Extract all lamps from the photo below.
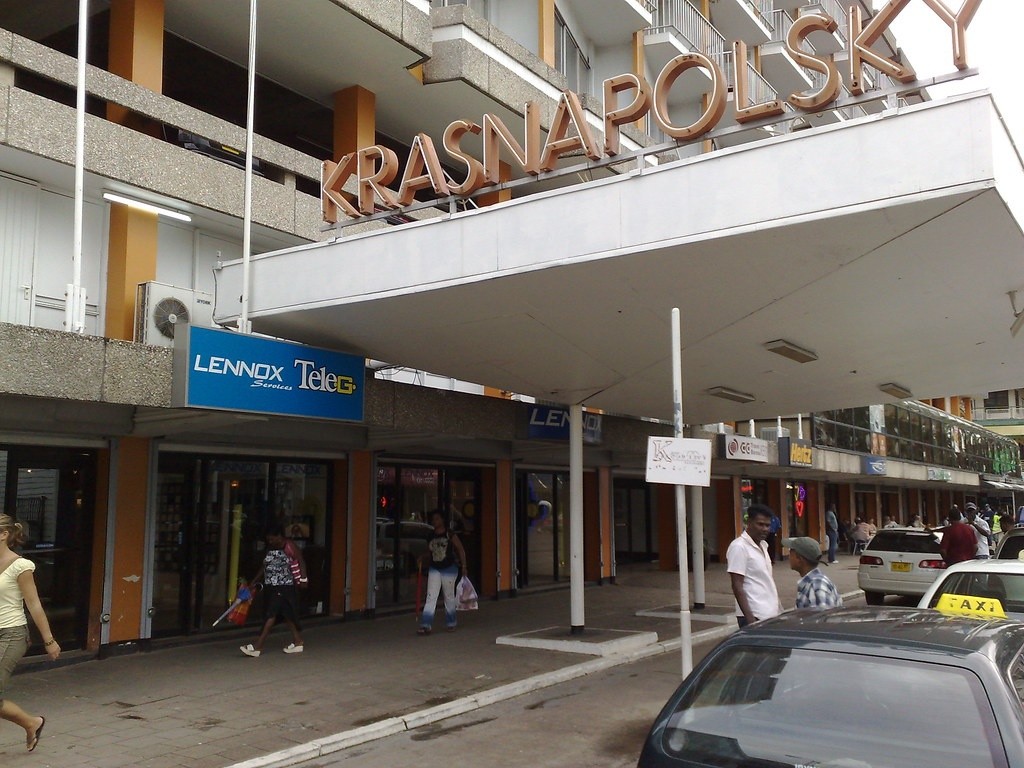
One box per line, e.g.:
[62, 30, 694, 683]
[707, 387, 756, 403]
[879, 383, 913, 399]
[764, 341, 817, 363]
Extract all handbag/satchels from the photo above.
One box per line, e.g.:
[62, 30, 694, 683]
[455, 577, 478, 610]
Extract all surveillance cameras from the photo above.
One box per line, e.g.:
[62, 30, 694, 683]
[1011, 308, 1024, 337]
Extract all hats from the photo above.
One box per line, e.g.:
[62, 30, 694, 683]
[964, 502, 976, 511]
[781, 537, 822, 563]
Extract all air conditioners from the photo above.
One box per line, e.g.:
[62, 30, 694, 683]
[760, 427, 791, 441]
[132, 280, 213, 349]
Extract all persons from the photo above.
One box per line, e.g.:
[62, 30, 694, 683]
[979, 502, 1004, 548]
[248, 526, 309, 659]
[727, 503, 784, 629]
[959, 501, 991, 561]
[937, 507, 979, 567]
[781, 537, 844, 610]
[824, 501, 839, 564]
[415, 510, 468, 637]
[912, 514, 929, 528]
[0, 510, 62, 754]
[883, 516, 900, 528]
[848, 515, 876, 554]
[998, 514, 1015, 534]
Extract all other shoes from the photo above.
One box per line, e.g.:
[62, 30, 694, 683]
[831, 561, 839, 564]
[416, 627, 431, 635]
[447, 626, 455, 633]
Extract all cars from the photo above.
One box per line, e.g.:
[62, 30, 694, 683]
[914, 523, 1024, 625]
[857, 525, 943, 606]
[637, 592, 1024, 768]
[374, 515, 434, 578]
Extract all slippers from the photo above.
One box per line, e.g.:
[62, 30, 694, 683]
[283, 643, 303, 653]
[27, 716, 44, 752]
[240, 644, 260, 657]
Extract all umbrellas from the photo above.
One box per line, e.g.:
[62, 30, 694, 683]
[212, 582, 264, 627]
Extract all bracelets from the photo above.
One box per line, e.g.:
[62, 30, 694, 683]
[299, 576, 307, 582]
[462, 564, 468, 567]
[44, 639, 55, 646]
